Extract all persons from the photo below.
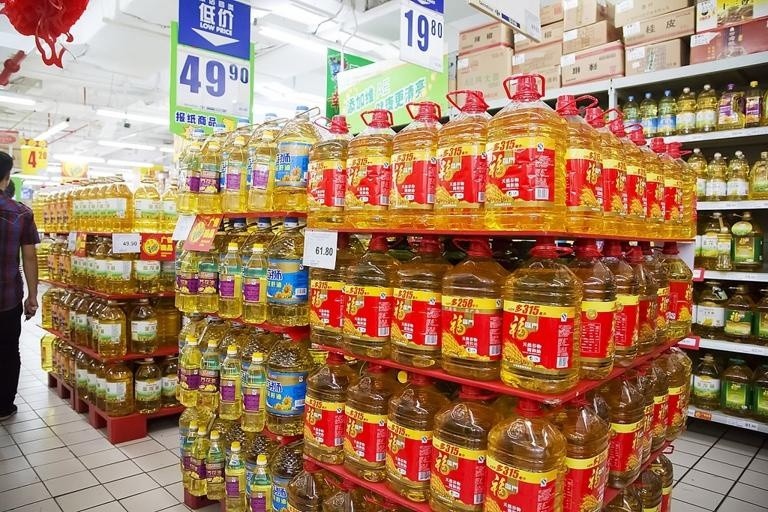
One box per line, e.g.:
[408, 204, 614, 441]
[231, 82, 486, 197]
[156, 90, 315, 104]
[4, 179, 16, 200]
[0, 151, 41, 422]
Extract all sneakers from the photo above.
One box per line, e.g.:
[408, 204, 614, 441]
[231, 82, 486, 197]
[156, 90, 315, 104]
[0, 404, 18, 420]
